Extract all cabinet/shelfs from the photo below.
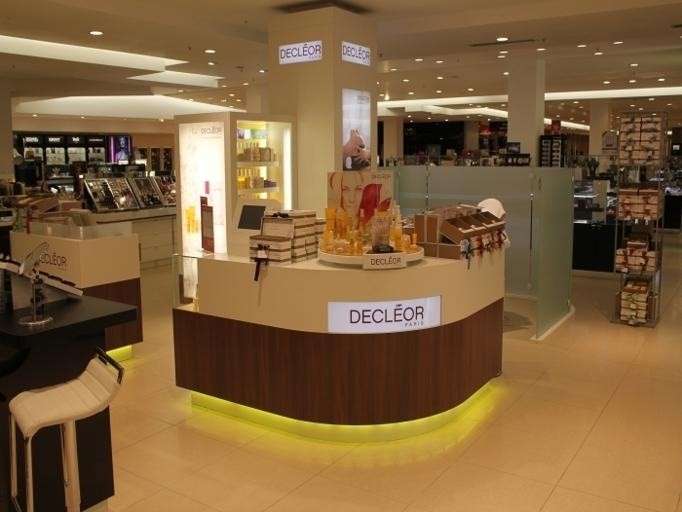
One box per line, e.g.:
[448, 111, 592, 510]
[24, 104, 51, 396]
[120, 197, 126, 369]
[612, 111, 669, 328]
[236, 122, 281, 206]
[126, 215, 177, 270]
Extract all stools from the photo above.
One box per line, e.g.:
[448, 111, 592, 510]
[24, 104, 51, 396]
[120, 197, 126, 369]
[6, 347, 125, 512]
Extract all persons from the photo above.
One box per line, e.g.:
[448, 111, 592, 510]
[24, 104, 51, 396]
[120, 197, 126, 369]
[115, 136, 129, 163]
[327, 171, 364, 215]
[351, 146, 371, 167]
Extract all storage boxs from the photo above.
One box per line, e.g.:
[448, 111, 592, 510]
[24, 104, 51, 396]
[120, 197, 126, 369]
[402, 205, 506, 260]
[249, 211, 326, 267]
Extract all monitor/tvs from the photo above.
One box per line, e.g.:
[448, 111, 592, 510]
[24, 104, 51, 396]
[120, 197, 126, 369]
[238, 205, 265, 230]
